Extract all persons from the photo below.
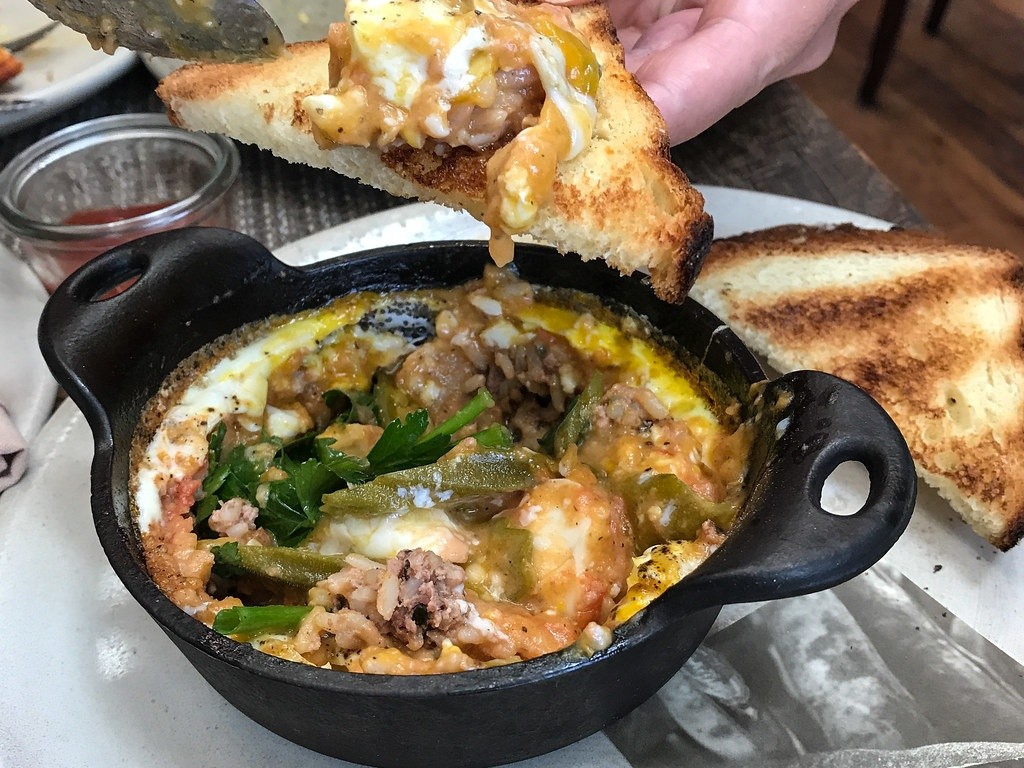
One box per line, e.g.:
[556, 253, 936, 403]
[545, 2, 864, 152]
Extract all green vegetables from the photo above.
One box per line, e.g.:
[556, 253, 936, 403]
[199, 393, 489, 544]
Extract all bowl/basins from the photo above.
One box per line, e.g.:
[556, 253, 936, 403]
[39, 224, 911, 768]
[0, 111, 241, 308]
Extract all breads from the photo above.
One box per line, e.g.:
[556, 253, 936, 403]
[684, 224, 1024, 549]
[156, 0, 710, 301]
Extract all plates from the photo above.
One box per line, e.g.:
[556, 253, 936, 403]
[137, 0, 351, 92]
[0, 186, 1024, 768]
[0, 0, 139, 132]
[0, 242, 60, 474]
